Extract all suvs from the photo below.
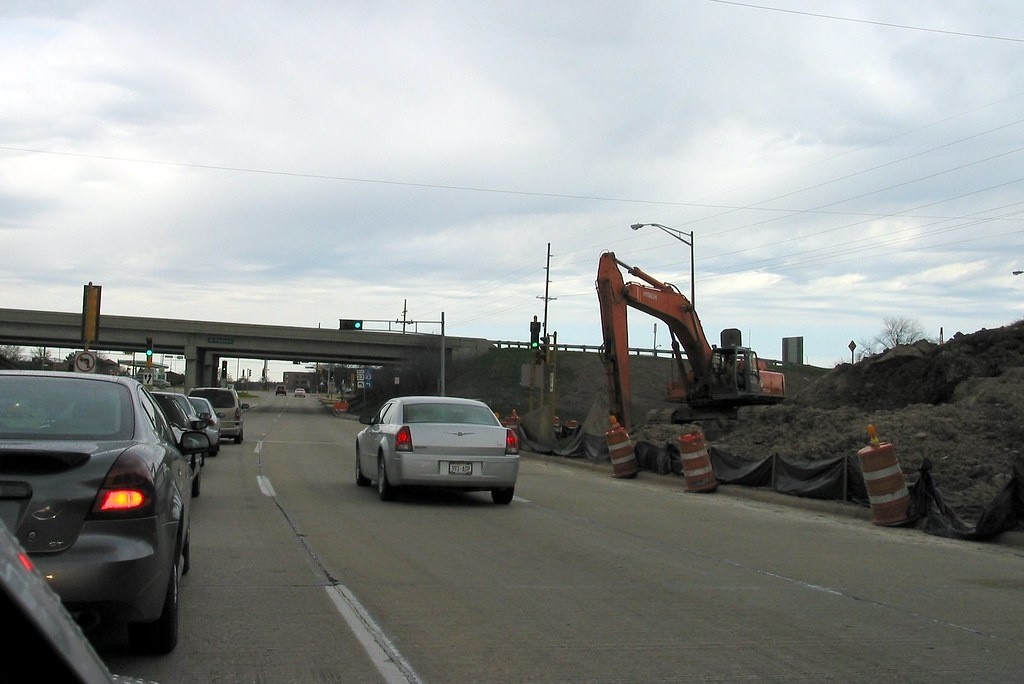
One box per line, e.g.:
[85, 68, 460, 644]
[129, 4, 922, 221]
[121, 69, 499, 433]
[183, 385, 250, 444]
[275, 385, 287, 396]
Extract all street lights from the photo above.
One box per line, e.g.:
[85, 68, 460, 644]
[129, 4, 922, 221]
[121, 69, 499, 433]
[631, 222, 694, 310]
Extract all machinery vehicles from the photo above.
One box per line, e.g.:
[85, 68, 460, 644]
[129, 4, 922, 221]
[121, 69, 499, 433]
[594, 248, 787, 442]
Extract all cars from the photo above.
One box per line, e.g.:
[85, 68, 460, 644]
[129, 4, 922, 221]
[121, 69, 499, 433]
[355, 395, 520, 504]
[149, 389, 226, 497]
[0, 370, 213, 657]
[294, 387, 306, 398]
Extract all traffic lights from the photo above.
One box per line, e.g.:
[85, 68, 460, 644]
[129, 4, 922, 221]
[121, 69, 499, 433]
[339, 319, 363, 331]
[530, 320, 541, 350]
[540, 333, 550, 351]
[146, 338, 153, 362]
[221, 361, 228, 378]
